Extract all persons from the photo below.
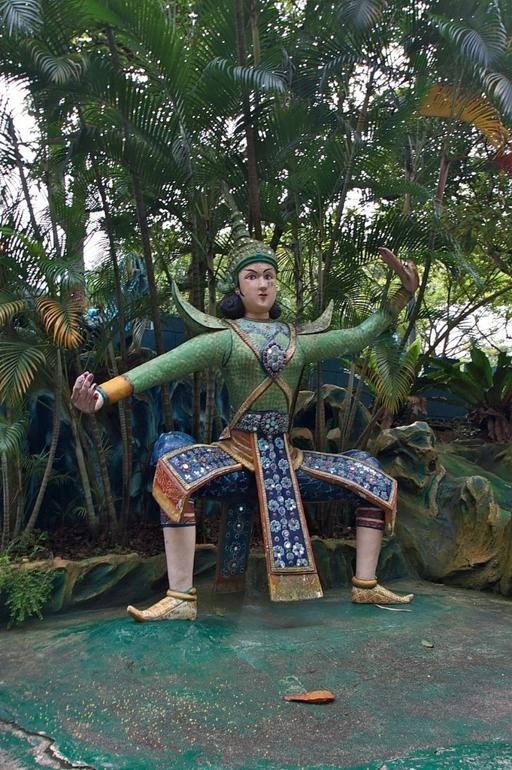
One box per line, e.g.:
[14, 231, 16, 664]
[68, 235, 421, 623]
